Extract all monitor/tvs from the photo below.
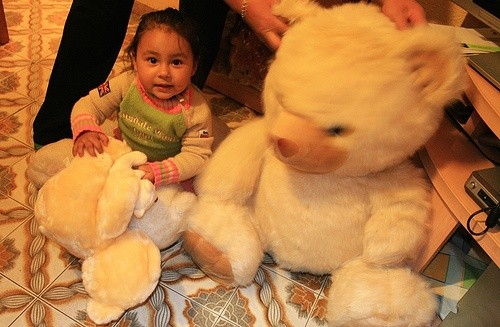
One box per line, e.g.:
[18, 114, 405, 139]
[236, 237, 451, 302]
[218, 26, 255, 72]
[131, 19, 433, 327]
[452, 0, 500, 90]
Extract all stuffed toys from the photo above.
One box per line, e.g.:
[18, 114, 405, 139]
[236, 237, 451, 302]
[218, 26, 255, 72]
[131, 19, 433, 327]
[30, 136, 198, 253]
[181, 0, 468, 327]
[29, 137, 201, 325]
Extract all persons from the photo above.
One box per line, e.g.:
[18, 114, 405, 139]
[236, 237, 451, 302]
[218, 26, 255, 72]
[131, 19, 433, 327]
[71, 9, 213, 184]
[33, 1, 427, 148]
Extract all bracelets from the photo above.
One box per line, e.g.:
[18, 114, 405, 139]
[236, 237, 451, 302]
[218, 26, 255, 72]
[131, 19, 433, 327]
[241, 0, 248, 22]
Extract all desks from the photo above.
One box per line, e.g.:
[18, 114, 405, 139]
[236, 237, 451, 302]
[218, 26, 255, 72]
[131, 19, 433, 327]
[414, 57, 500, 276]
